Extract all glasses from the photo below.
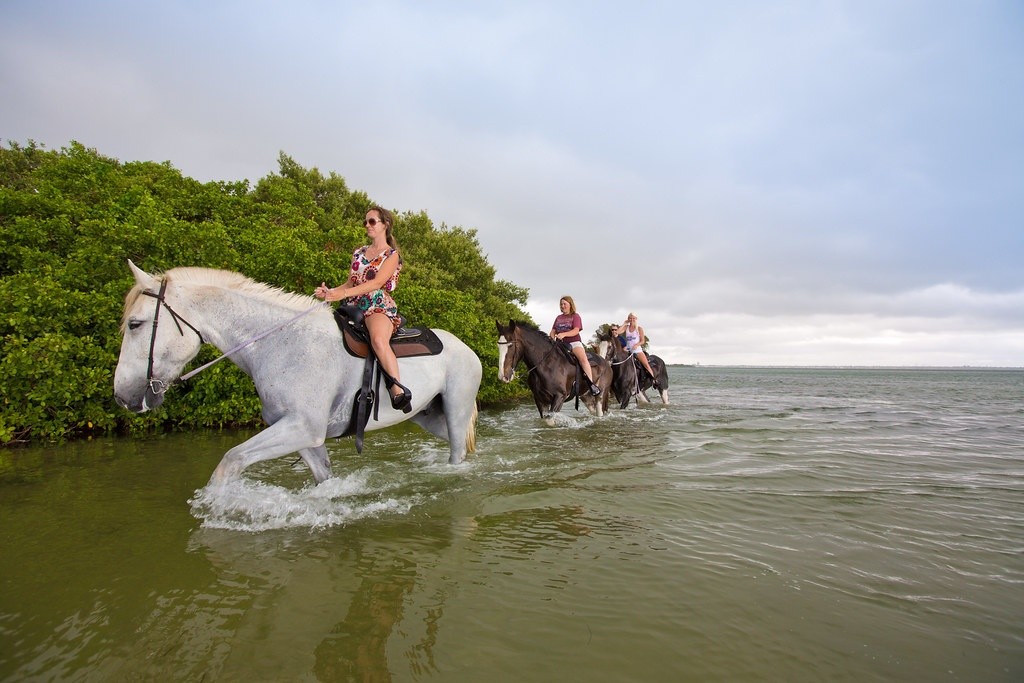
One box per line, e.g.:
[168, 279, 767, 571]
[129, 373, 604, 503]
[363, 218, 381, 227]
[612, 329, 617, 331]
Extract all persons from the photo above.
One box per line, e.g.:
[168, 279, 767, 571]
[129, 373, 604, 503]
[551, 296, 599, 395]
[315, 207, 412, 415]
[611, 324, 626, 350]
[617, 313, 654, 378]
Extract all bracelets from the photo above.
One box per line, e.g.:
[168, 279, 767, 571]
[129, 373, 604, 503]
[344, 289, 348, 298]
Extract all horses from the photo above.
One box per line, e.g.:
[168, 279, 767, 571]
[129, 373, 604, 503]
[113, 259, 484, 491]
[492, 318, 670, 422]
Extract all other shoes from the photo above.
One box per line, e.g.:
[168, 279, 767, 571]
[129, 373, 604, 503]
[394, 393, 412, 414]
[591, 384, 600, 395]
[652, 379, 660, 390]
